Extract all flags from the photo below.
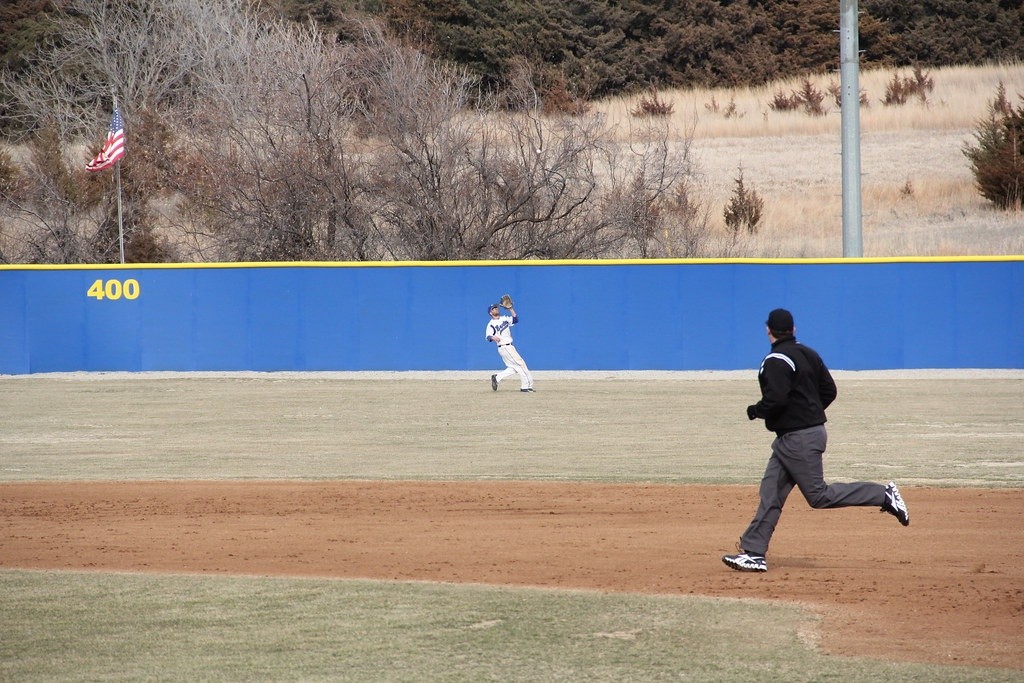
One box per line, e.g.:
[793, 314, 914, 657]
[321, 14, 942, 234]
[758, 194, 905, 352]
[85, 107, 125, 171]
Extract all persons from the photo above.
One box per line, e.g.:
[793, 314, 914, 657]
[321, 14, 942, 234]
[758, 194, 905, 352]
[721, 308, 909, 572]
[486, 294, 536, 392]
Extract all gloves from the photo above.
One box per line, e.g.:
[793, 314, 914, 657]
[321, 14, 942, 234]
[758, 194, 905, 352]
[747, 405, 757, 420]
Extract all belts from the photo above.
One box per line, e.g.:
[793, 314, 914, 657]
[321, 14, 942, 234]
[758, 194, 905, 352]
[499, 343, 512, 347]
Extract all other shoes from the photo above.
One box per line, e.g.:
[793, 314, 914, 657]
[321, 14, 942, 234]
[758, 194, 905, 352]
[521, 388, 535, 392]
[492, 374, 498, 391]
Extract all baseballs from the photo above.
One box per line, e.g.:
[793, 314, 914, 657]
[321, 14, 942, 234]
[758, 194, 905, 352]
[535, 148, 541, 155]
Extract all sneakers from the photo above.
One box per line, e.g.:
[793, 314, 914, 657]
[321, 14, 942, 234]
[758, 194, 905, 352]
[722, 542, 767, 572]
[879, 482, 909, 526]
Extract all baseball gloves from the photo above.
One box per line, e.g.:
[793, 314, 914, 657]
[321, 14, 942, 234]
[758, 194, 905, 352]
[499, 294, 513, 310]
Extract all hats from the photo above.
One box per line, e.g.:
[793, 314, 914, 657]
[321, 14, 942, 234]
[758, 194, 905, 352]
[488, 304, 498, 314]
[766, 309, 794, 331]
